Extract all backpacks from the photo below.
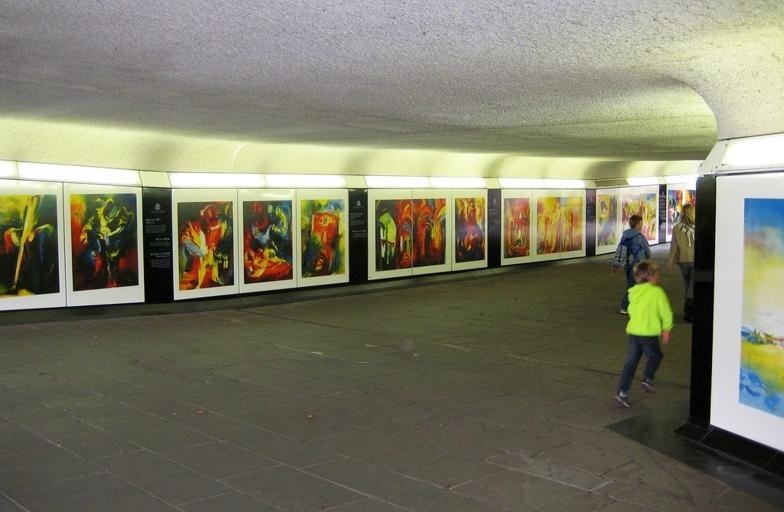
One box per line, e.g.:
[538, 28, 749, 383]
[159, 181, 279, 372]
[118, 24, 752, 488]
[614, 238, 637, 268]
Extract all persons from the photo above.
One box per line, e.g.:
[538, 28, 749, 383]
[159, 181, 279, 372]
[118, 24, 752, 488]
[610, 214, 651, 316]
[666, 203, 695, 324]
[613, 258, 674, 408]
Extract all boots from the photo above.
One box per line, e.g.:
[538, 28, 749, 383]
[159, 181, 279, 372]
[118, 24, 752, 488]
[684, 297, 694, 321]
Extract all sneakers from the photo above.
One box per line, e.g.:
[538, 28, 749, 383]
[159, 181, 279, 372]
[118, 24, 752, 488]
[641, 378, 654, 391]
[620, 309, 627, 313]
[615, 391, 631, 408]
